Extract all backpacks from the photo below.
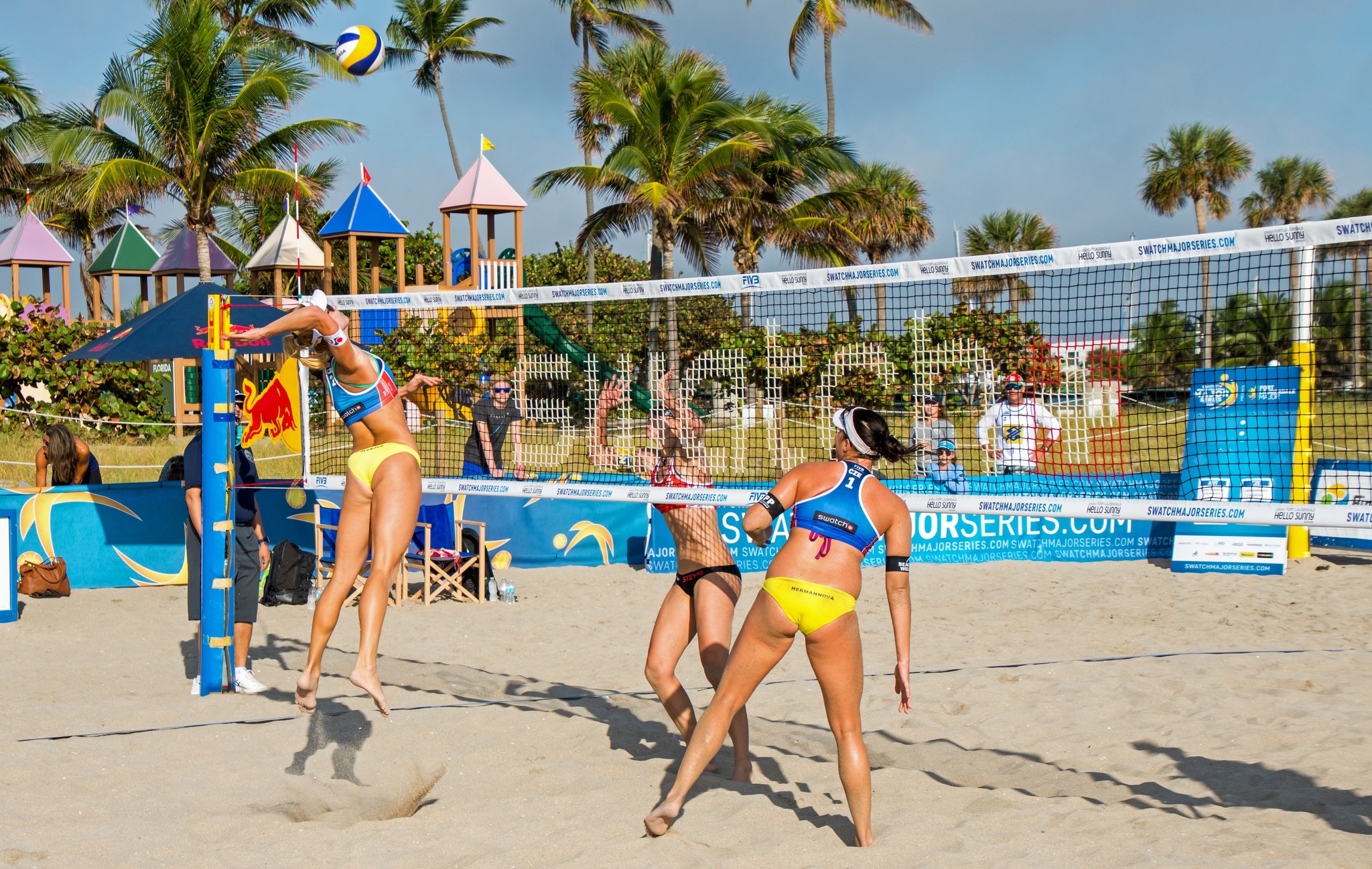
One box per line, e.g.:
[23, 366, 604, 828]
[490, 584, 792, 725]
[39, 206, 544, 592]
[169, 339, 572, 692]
[461, 527, 500, 599]
[261, 540, 316, 607]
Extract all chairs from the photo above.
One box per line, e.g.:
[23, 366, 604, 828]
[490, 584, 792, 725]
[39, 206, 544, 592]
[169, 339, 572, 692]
[399, 502, 485, 605]
[313, 504, 402, 608]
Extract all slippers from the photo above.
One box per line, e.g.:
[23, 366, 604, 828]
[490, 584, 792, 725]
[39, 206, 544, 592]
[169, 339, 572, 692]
[29, 588, 70, 598]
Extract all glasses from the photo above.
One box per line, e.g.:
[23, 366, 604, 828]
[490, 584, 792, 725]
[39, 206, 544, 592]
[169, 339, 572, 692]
[925, 401, 937, 404]
[938, 450, 951, 456]
[235, 400, 244, 409]
[327, 304, 335, 314]
[42, 436, 49, 446]
[650, 405, 677, 417]
[494, 387, 511, 393]
[1006, 385, 1021, 390]
[841, 405, 864, 447]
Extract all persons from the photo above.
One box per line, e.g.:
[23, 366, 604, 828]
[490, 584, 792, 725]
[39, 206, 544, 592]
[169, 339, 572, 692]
[35, 427, 105, 490]
[640, 406, 913, 848]
[975, 373, 1062, 473]
[463, 379, 530, 480]
[180, 400, 271, 696]
[909, 394, 966, 480]
[225, 307, 421, 717]
[591, 379, 754, 785]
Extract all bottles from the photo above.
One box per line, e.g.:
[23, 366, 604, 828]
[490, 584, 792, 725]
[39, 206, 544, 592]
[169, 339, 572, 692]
[500, 577, 506, 602]
[318, 587, 325, 600]
[506, 579, 515, 604]
[316, 585, 322, 606]
[306, 584, 316, 610]
[488, 577, 497, 601]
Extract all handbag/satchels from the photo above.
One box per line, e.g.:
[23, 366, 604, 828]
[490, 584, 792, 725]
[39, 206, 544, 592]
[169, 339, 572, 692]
[18, 555, 71, 597]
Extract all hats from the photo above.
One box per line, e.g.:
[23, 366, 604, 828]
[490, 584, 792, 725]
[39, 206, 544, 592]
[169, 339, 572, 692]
[937, 441, 955, 451]
[1005, 374, 1025, 386]
[235, 389, 246, 399]
[832, 406, 881, 455]
[923, 393, 942, 405]
[297, 289, 327, 348]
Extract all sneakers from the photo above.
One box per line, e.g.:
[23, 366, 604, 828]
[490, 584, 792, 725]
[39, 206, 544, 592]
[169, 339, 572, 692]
[235, 668, 268, 693]
[191, 677, 201, 695]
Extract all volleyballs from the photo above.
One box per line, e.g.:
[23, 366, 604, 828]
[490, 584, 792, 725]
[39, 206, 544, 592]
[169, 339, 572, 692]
[333, 24, 386, 77]
